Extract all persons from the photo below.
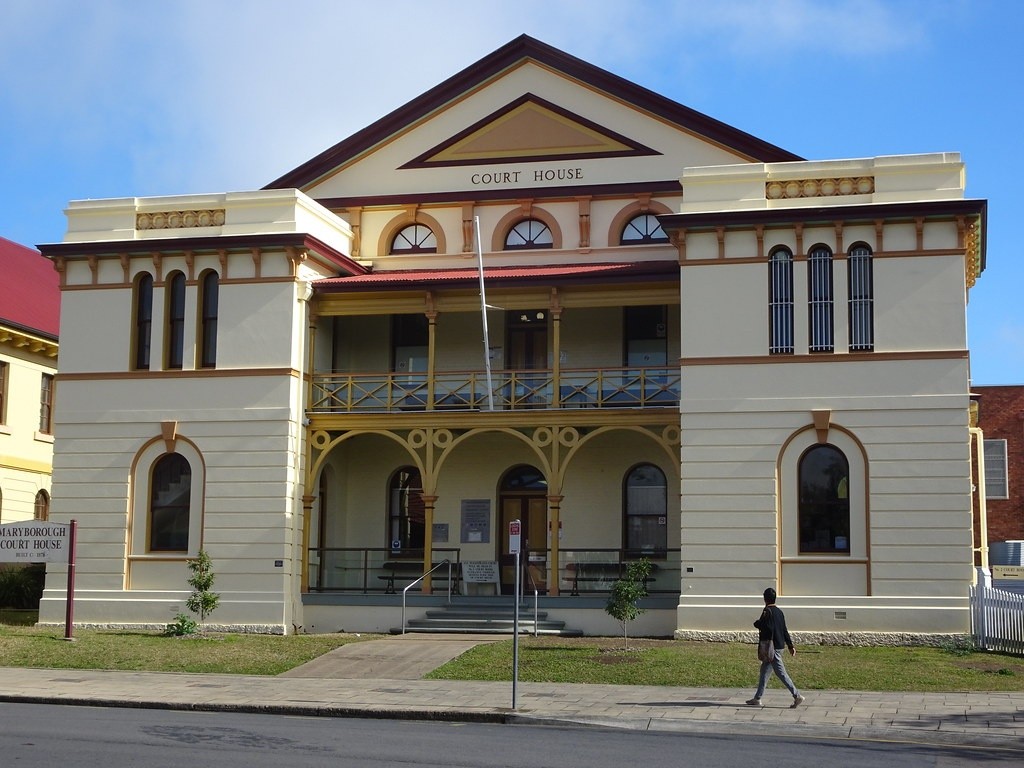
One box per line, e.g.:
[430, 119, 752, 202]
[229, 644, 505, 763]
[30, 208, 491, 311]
[746, 588, 805, 709]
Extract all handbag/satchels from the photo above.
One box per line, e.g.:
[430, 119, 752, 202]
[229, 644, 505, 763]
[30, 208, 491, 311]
[758, 640, 774, 663]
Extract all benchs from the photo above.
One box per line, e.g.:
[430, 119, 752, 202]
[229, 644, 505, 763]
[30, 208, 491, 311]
[561, 562, 660, 596]
[591, 389, 677, 408]
[377, 562, 464, 597]
[398, 392, 481, 409]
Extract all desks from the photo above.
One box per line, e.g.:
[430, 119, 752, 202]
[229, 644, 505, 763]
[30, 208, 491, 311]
[559, 385, 590, 408]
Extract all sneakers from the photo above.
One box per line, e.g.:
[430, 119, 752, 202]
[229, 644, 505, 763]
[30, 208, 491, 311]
[790, 696, 805, 709]
[746, 699, 762, 705]
